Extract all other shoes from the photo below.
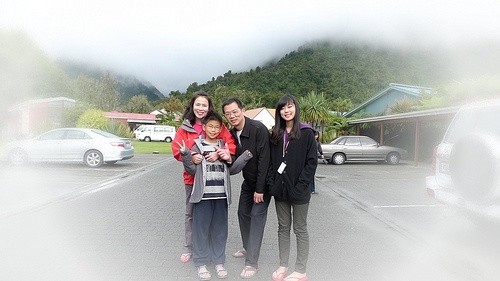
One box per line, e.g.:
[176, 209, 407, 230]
[181, 253, 190, 262]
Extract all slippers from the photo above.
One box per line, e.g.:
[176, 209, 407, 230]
[233, 248, 247, 258]
[284, 273, 307, 281]
[241, 266, 258, 278]
[272, 267, 288, 281]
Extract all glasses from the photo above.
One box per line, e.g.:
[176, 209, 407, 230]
[224, 109, 242, 118]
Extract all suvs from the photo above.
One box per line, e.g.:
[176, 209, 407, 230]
[421, 97, 500, 230]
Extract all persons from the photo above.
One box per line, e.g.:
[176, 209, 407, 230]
[172, 90, 236, 263]
[311, 131, 324, 193]
[177, 114, 252, 281]
[213, 98, 271, 279]
[266, 94, 318, 281]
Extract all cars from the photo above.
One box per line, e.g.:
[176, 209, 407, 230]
[3, 126, 135, 168]
[317, 136, 408, 165]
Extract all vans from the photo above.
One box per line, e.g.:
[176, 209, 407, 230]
[133, 124, 176, 143]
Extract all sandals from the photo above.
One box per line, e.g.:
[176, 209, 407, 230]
[216, 264, 227, 278]
[198, 267, 211, 280]
